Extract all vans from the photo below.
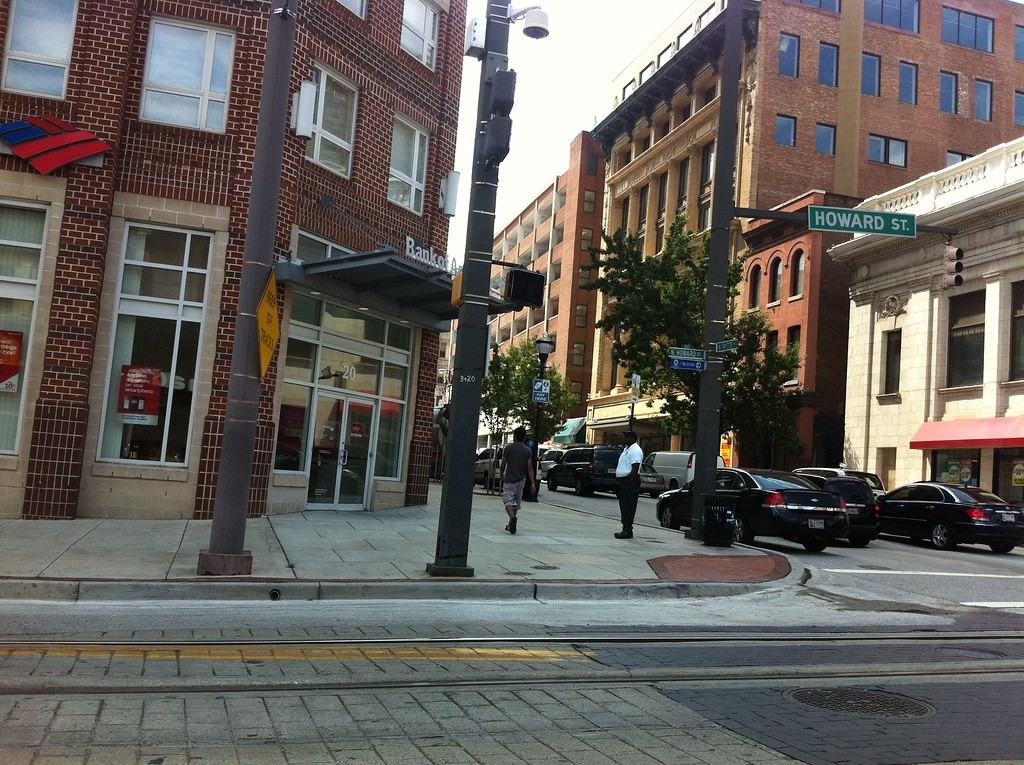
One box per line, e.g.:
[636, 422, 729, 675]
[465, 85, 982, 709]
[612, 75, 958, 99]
[643, 451, 725, 490]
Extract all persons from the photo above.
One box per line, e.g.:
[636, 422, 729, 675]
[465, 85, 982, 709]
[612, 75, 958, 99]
[614, 431, 643, 539]
[500, 428, 536, 536]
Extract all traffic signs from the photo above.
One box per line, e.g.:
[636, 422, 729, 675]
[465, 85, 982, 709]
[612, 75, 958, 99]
[668, 339, 738, 371]
[809, 204, 918, 238]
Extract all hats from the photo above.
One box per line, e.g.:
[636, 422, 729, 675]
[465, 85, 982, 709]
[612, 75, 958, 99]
[623, 431, 638, 440]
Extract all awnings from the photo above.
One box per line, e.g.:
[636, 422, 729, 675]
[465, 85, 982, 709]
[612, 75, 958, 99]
[909, 416, 1024, 448]
[553, 417, 585, 443]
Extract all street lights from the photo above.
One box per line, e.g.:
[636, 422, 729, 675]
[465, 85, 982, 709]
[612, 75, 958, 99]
[526, 331, 554, 502]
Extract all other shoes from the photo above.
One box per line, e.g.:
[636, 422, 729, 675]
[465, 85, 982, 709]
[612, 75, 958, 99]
[510, 517, 517, 534]
[505, 525, 510, 531]
[615, 531, 633, 539]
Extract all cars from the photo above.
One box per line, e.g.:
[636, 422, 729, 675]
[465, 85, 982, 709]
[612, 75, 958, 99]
[538, 449, 569, 479]
[474, 446, 543, 491]
[794, 471, 880, 548]
[655, 466, 849, 553]
[640, 464, 667, 497]
[877, 481, 1024, 554]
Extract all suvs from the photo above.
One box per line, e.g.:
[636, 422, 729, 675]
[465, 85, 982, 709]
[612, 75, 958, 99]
[547, 444, 624, 495]
[792, 467, 887, 499]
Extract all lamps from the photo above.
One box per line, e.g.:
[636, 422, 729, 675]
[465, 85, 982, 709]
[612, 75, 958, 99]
[290, 78, 317, 140]
[439, 169, 459, 217]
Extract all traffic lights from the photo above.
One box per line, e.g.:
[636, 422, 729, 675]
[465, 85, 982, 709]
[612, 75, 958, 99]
[504, 267, 544, 308]
[945, 246, 963, 289]
